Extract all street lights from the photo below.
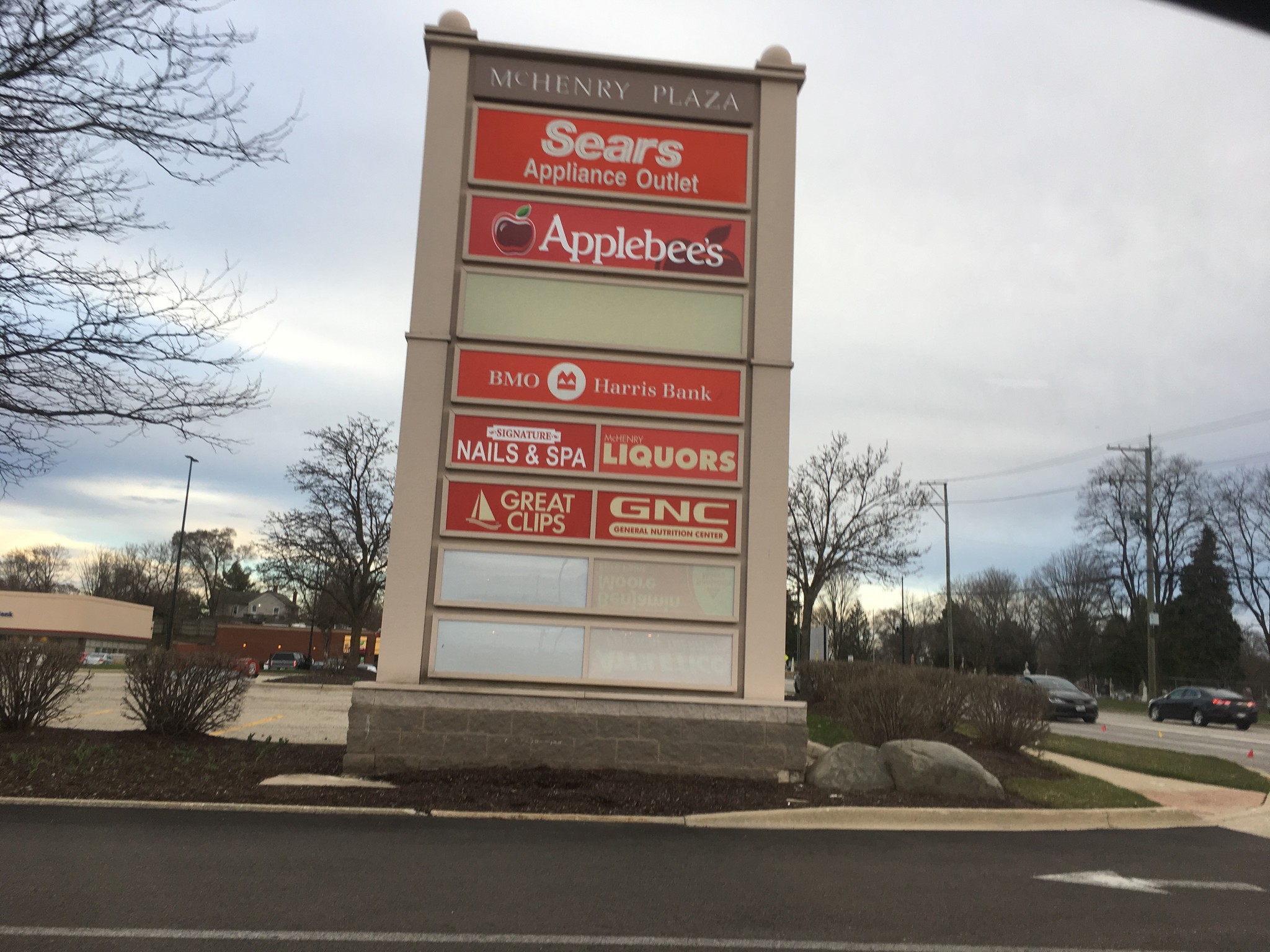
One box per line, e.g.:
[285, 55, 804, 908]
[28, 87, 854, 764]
[165, 454, 199, 652]
[308, 542, 325, 656]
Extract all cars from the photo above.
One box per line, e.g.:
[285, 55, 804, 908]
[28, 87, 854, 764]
[264, 651, 377, 674]
[999, 674, 1099, 724]
[69, 652, 113, 666]
[1147, 685, 1260, 731]
